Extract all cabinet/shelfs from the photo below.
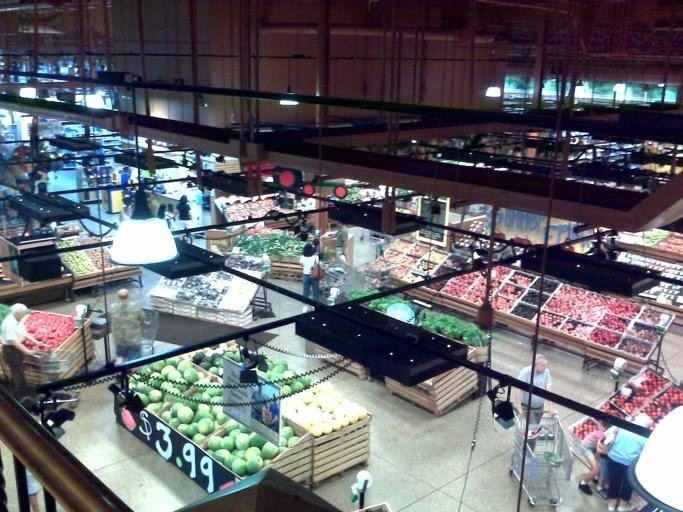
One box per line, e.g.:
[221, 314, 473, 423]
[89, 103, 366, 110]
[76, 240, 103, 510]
[117, 339, 373, 491]
[385, 330, 489, 416]
[305, 287, 413, 381]
[372, 237, 676, 376]
[233, 226, 318, 276]
[55, 238, 144, 302]
[567, 367, 683, 475]
[0, 303, 95, 387]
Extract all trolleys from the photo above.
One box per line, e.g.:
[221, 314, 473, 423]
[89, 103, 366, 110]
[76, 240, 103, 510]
[507, 412, 574, 512]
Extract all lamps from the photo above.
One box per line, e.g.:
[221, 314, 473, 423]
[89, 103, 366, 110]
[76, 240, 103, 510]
[19, 59, 36, 98]
[108, 89, 179, 266]
[280, 58, 299, 105]
[486, 61, 501, 97]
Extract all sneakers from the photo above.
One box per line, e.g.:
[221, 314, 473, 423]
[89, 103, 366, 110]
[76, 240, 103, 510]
[607, 503, 616, 511]
[616, 504, 635, 511]
[579, 481, 594, 495]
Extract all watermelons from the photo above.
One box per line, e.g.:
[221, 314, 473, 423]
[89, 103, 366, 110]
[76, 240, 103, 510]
[126, 349, 312, 479]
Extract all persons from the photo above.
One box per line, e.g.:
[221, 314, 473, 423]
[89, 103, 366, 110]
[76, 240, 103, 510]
[106, 288, 147, 362]
[0, 302, 47, 401]
[515, 354, 557, 457]
[578, 413, 652, 512]
[586, 242, 610, 261]
[250, 384, 279, 433]
[299, 221, 348, 302]
[112, 165, 192, 230]
[429, 195, 441, 224]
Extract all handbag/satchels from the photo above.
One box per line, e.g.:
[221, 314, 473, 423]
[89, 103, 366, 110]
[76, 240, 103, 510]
[311, 261, 325, 279]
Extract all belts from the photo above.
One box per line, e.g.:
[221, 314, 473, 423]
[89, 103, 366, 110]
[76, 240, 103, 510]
[336, 246, 343, 247]
[522, 403, 544, 410]
